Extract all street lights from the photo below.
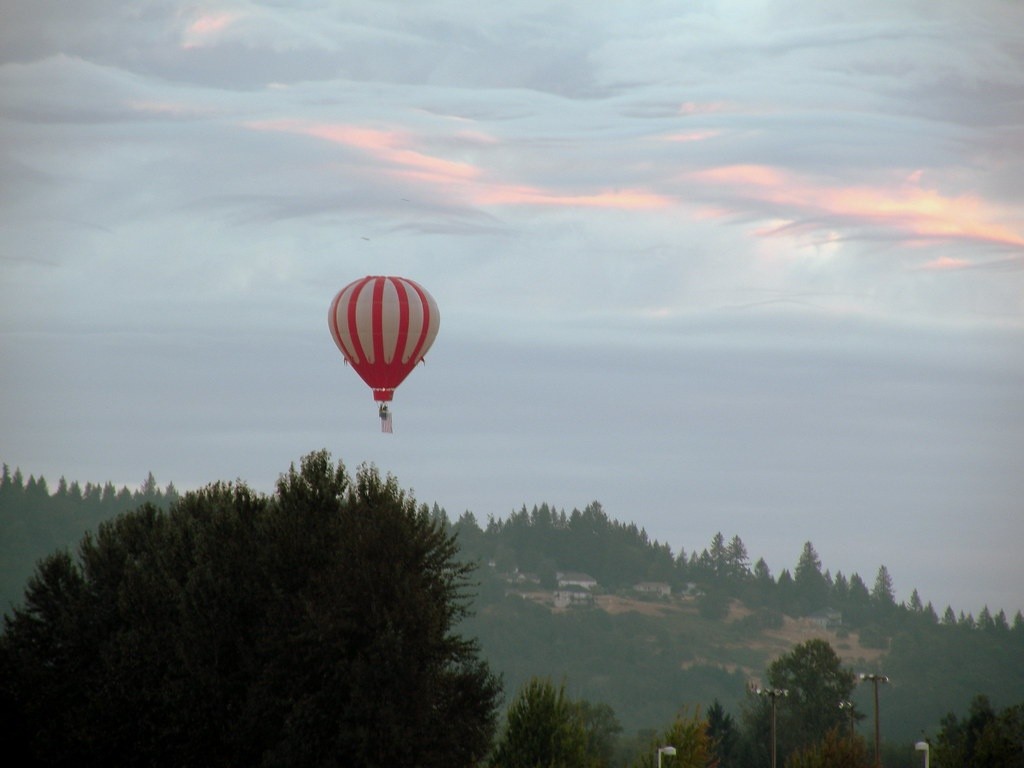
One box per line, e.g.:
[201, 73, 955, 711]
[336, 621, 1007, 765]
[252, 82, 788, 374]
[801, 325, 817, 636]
[656, 746, 677, 768]
[916, 740, 930, 768]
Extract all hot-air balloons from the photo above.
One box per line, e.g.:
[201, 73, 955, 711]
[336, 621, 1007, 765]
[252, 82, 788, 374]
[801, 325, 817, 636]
[326, 273, 441, 420]
